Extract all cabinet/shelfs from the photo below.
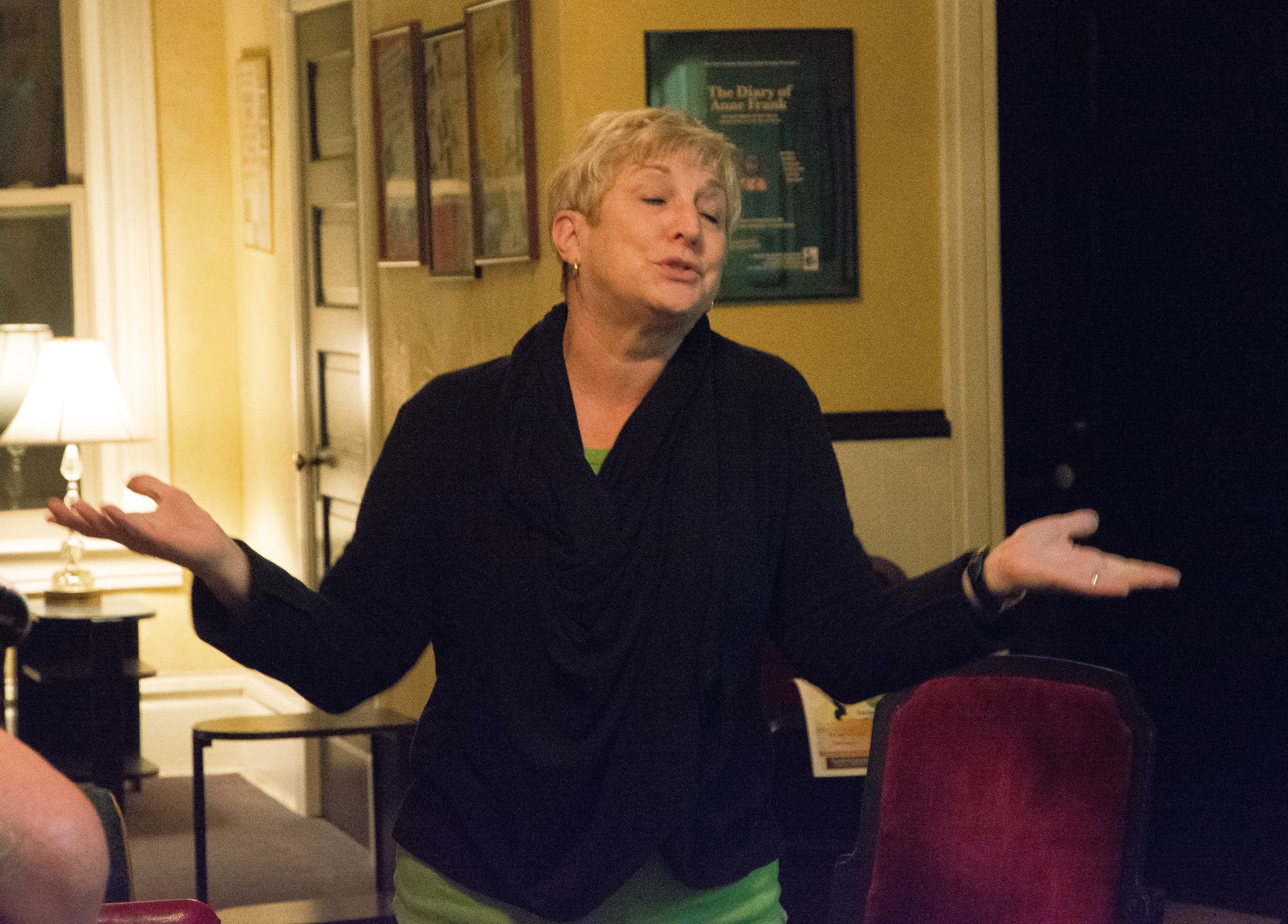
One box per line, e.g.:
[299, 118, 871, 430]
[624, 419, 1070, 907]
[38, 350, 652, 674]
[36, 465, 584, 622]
[9, 597, 158, 815]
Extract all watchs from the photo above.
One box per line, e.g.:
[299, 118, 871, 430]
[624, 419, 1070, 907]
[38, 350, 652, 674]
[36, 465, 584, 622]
[966, 542, 1027, 615]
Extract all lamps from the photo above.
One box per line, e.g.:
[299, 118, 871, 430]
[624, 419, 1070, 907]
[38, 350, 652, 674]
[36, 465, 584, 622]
[0, 322, 58, 543]
[0, 335, 157, 602]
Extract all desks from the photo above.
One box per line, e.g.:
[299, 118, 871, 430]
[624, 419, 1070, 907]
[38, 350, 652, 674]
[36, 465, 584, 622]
[187, 709, 415, 924]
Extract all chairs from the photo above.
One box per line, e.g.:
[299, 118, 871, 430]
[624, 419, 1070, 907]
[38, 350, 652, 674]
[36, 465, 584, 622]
[828, 653, 1169, 924]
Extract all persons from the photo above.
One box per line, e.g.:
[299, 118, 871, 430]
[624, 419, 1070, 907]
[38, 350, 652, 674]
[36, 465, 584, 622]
[44, 103, 1181, 921]
[1, 728, 110, 923]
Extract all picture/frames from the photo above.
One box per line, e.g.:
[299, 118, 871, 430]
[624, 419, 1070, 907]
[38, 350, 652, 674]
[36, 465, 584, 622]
[462, 0, 540, 263]
[365, 19, 428, 270]
[418, 20, 482, 282]
[231, 52, 276, 254]
[643, 26, 858, 304]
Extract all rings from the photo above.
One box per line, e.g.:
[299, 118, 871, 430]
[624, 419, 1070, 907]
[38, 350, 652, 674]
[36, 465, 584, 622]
[1088, 570, 1099, 586]
